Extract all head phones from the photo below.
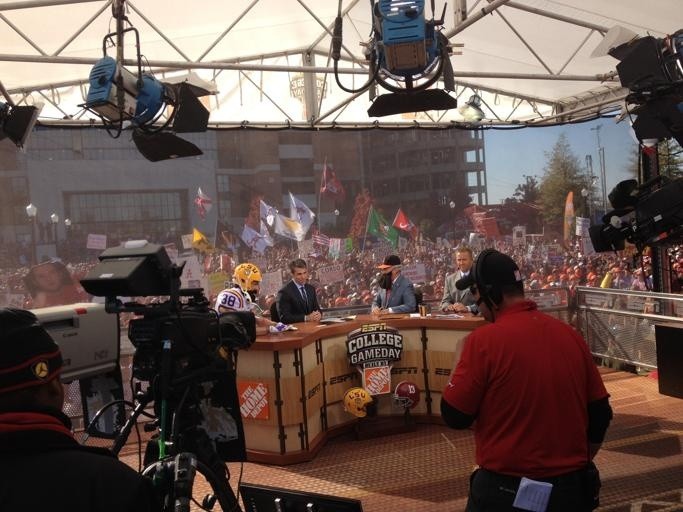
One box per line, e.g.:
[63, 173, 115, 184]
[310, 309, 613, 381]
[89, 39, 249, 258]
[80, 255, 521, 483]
[471, 247, 505, 310]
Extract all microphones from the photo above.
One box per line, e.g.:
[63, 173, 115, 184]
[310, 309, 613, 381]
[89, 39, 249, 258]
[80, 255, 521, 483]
[466, 295, 484, 318]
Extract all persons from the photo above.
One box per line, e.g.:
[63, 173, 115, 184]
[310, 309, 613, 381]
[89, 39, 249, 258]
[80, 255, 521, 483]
[1, 256, 193, 330]
[1, 308, 165, 512]
[441, 248, 612, 512]
[197, 237, 682, 374]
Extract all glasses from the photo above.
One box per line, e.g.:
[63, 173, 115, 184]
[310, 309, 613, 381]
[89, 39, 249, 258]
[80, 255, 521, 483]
[470, 287, 478, 297]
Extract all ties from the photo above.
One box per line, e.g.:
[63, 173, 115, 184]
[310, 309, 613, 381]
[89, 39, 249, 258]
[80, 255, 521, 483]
[386, 289, 391, 308]
[299, 287, 307, 313]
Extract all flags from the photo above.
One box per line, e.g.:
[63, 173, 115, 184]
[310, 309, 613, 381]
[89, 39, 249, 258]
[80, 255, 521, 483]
[366, 207, 398, 249]
[320, 161, 346, 201]
[311, 224, 330, 246]
[195, 188, 212, 212]
[260, 220, 276, 246]
[288, 193, 315, 232]
[241, 226, 266, 253]
[214, 220, 240, 254]
[274, 214, 304, 242]
[465, 207, 497, 238]
[192, 228, 207, 242]
[392, 208, 417, 239]
[259, 200, 278, 226]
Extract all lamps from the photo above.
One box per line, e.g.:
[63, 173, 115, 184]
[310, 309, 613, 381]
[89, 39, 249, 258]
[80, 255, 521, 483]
[459, 95, 484, 122]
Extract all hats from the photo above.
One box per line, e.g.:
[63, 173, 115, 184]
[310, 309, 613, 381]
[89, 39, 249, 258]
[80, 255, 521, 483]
[377, 255, 400, 268]
[456, 253, 522, 291]
[0, 309, 62, 395]
[530, 257, 646, 290]
[23, 256, 69, 299]
[315, 272, 380, 306]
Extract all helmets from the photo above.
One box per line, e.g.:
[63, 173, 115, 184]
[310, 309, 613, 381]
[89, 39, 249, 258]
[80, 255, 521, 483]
[392, 381, 420, 410]
[232, 263, 263, 291]
[342, 387, 373, 417]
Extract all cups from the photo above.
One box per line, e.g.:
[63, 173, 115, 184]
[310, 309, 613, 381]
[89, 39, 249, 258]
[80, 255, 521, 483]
[418, 304, 428, 318]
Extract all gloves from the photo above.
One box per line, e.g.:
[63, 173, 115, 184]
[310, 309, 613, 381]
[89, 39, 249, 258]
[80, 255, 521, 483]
[276, 322, 298, 330]
[269, 323, 294, 333]
